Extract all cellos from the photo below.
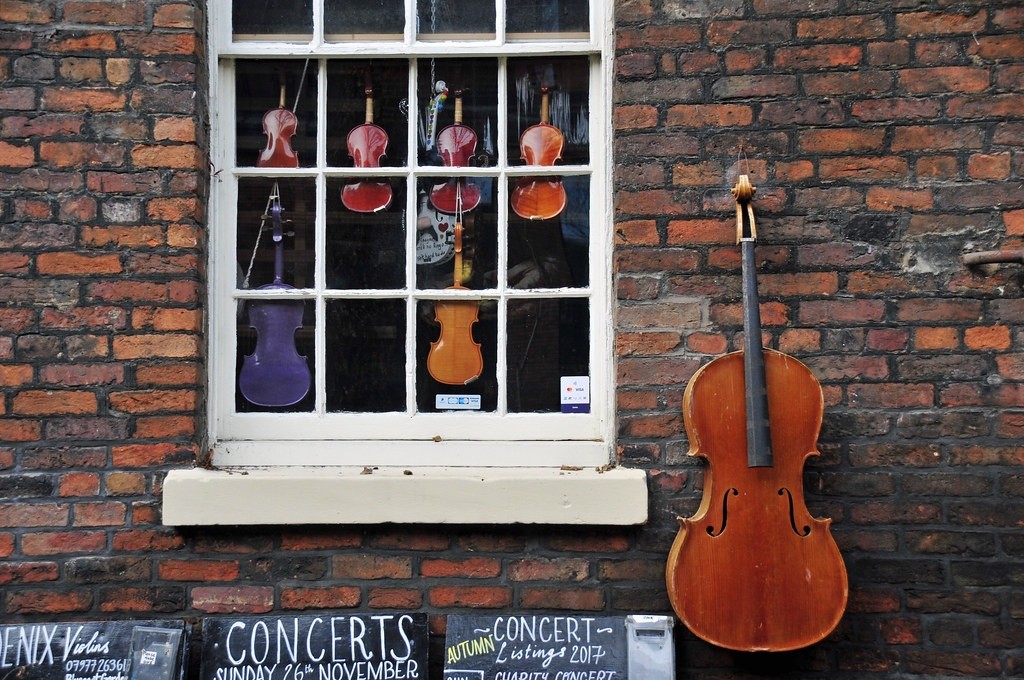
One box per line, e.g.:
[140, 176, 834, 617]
[664, 173, 847, 652]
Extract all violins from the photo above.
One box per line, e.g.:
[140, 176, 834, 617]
[511, 81, 569, 221]
[429, 87, 481, 215]
[339, 70, 394, 213]
[257, 66, 301, 168]
[426, 221, 482, 383]
[239, 194, 311, 407]
